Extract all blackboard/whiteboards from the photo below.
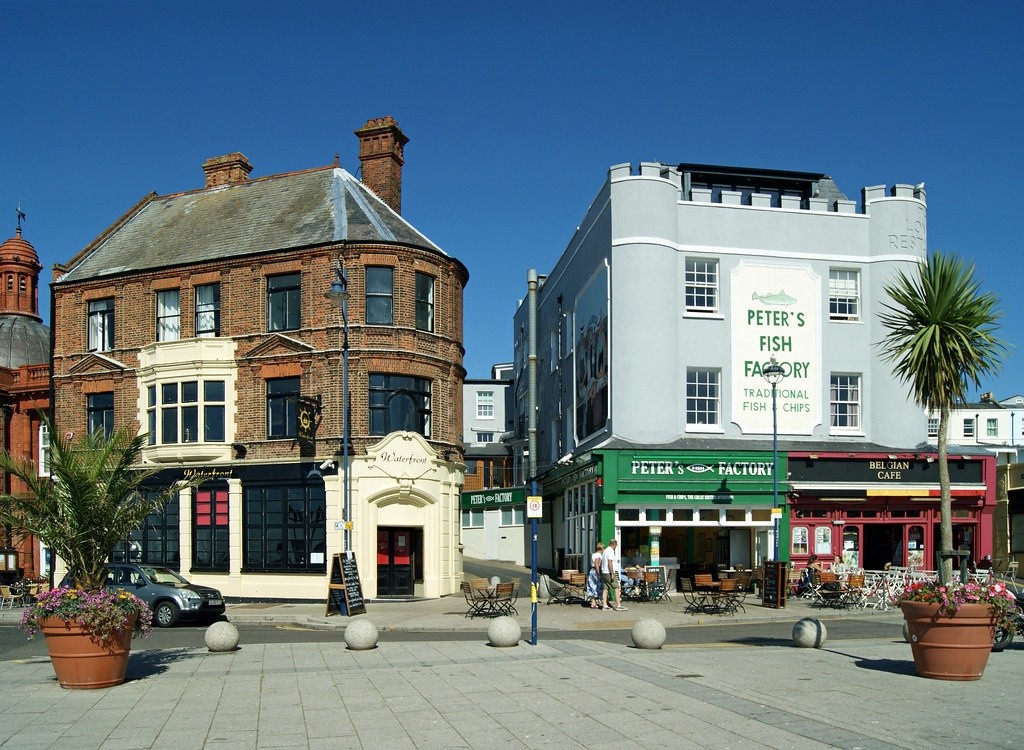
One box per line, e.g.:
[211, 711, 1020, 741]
[326, 552, 368, 610]
[762, 562, 781, 605]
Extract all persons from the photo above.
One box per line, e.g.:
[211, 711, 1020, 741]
[804, 554, 824, 595]
[600, 539, 629, 611]
[586, 543, 605, 608]
[976, 553, 993, 570]
[833, 554, 846, 579]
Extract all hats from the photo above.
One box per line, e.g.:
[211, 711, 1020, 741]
[597, 543, 605, 549]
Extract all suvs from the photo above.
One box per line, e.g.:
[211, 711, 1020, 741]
[57, 562, 226, 627]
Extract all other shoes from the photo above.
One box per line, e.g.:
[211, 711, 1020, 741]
[602, 606, 613, 611]
[616, 606, 629, 610]
[591, 605, 603, 609]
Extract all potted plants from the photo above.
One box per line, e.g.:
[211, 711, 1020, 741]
[873, 249, 1020, 681]
[0, 395, 215, 689]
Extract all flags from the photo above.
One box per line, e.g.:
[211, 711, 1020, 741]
[295, 400, 315, 447]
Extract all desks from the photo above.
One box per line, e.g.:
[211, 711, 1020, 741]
[836, 580, 849, 608]
[721, 569, 752, 593]
[867, 577, 902, 608]
[971, 574, 990, 584]
[557, 576, 570, 604]
[694, 582, 722, 613]
[472, 586, 508, 617]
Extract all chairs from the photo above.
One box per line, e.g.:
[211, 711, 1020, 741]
[0, 585, 26, 610]
[680, 562, 1019, 618]
[542, 568, 675, 606]
[461, 577, 521, 620]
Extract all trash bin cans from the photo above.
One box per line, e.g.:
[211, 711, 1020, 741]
[555, 548, 571, 574]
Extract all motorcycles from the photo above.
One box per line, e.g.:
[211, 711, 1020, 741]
[990, 585, 1024, 650]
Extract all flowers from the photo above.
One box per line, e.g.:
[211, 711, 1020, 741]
[893, 578, 1020, 642]
[18, 583, 154, 641]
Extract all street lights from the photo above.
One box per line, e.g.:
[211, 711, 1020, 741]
[764, 353, 785, 561]
[325, 259, 352, 549]
[527, 268, 547, 644]
